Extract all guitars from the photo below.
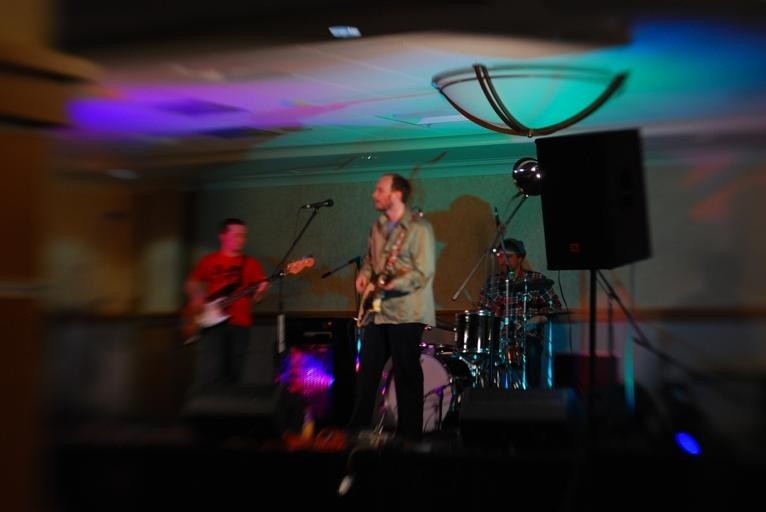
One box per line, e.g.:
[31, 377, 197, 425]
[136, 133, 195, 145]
[193, 257, 316, 330]
[356, 265, 409, 329]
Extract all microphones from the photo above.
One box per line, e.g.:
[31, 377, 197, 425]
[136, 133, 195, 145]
[302, 199, 333, 209]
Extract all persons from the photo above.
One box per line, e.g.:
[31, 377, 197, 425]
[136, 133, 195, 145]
[343, 173, 437, 442]
[180, 217, 272, 401]
[473, 238, 562, 388]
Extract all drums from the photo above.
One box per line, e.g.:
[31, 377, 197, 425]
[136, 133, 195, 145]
[494, 341, 524, 371]
[463, 307, 502, 362]
[454, 310, 465, 355]
[380, 345, 484, 439]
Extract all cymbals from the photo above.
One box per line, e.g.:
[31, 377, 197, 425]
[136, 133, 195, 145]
[531, 306, 575, 318]
[510, 299, 553, 306]
[503, 279, 555, 295]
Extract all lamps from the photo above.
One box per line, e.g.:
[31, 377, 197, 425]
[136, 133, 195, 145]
[429, 65, 632, 139]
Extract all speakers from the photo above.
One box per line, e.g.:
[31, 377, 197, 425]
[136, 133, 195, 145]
[181, 394, 303, 448]
[536, 129, 652, 271]
[457, 388, 571, 449]
